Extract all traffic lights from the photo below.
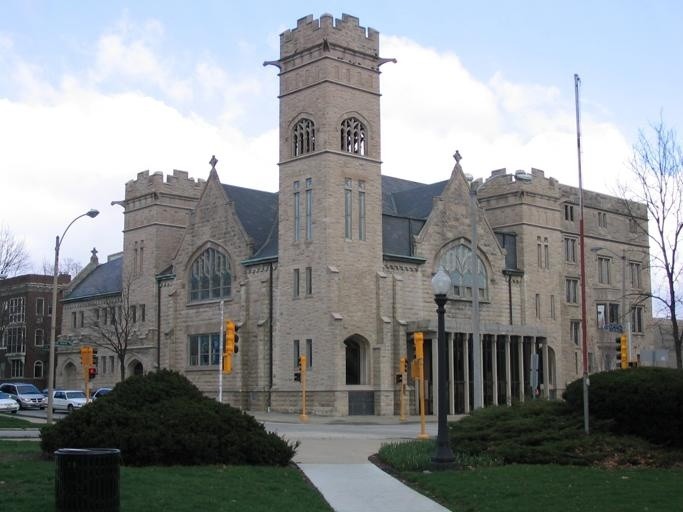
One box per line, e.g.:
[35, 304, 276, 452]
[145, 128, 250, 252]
[408, 333, 419, 360]
[88, 368, 97, 379]
[293, 372, 301, 381]
[89, 348, 98, 365]
[400, 357, 408, 373]
[225, 320, 240, 355]
[615, 335, 628, 370]
[298, 355, 306, 371]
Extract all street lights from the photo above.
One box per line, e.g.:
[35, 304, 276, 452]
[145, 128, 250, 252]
[429, 264, 458, 471]
[46, 209, 101, 429]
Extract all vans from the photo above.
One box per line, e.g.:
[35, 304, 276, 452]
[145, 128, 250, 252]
[0, 382, 46, 411]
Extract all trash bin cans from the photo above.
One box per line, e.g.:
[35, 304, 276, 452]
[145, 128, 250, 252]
[54, 448, 123, 512]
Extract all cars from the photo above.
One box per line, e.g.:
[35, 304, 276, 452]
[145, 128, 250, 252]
[41, 389, 94, 415]
[0, 391, 20, 415]
[40, 387, 59, 399]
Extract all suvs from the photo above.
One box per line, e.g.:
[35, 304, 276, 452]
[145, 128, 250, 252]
[92, 388, 113, 402]
